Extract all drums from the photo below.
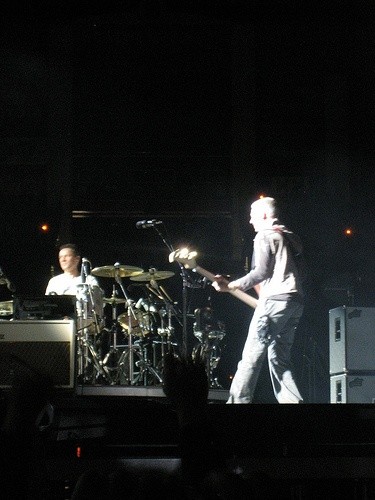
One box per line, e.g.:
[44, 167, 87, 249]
[193, 319, 226, 338]
[193, 342, 234, 390]
[63, 282, 105, 334]
[117, 310, 154, 336]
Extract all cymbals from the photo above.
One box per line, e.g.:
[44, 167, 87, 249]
[103, 298, 134, 304]
[130, 271, 175, 282]
[91, 265, 143, 277]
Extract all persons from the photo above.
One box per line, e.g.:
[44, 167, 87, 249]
[45, 243, 103, 317]
[213, 196, 307, 405]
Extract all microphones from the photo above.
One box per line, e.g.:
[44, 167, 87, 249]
[135, 219, 163, 229]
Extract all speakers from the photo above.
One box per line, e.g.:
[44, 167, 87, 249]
[328, 306, 375, 404]
[0, 319, 75, 388]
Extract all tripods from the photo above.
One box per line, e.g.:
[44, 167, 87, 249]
[76, 228, 188, 388]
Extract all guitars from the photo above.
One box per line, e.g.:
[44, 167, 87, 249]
[169, 248, 259, 310]
